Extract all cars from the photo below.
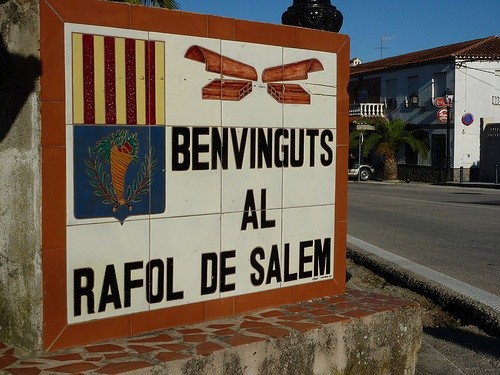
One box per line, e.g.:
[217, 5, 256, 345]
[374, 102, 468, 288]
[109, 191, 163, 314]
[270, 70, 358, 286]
[347, 156, 376, 181]
